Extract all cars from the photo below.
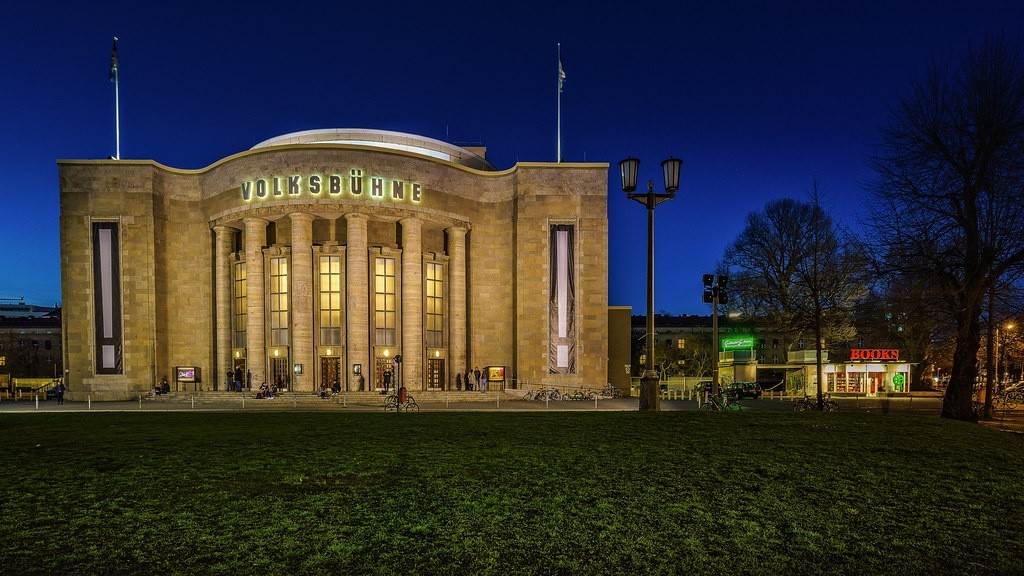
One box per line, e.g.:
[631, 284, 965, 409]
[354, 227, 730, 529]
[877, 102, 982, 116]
[1000, 379, 1024, 398]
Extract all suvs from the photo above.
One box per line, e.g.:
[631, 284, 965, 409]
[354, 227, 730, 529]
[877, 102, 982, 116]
[725, 382, 763, 400]
[691, 380, 722, 398]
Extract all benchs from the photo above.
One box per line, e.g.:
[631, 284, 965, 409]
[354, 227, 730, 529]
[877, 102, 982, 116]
[19, 390, 47, 401]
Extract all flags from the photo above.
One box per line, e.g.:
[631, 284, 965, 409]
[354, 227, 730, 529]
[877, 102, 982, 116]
[556, 44, 565, 93]
[109, 38, 119, 82]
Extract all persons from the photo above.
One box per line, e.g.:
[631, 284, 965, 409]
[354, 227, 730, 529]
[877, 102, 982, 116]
[455, 367, 488, 392]
[55, 381, 65, 405]
[384, 369, 392, 391]
[155, 380, 170, 396]
[225, 365, 341, 400]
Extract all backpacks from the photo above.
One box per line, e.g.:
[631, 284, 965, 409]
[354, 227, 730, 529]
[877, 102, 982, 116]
[256, 393, 261, 399]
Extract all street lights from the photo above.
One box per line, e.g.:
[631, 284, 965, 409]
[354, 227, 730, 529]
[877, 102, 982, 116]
[392, 354, 403, 395]
[994, 322, 1015, 394]
[700, 273, 729, 411]
[619, 155, 684, 412]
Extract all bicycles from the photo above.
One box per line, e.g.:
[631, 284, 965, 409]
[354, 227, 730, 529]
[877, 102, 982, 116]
[701, 393, 743, 412]
[521, 382, 624, 401]
[792, 390, 840, 414]
[382, 388, 421, 413]
[991, 391, 1024, 409]
